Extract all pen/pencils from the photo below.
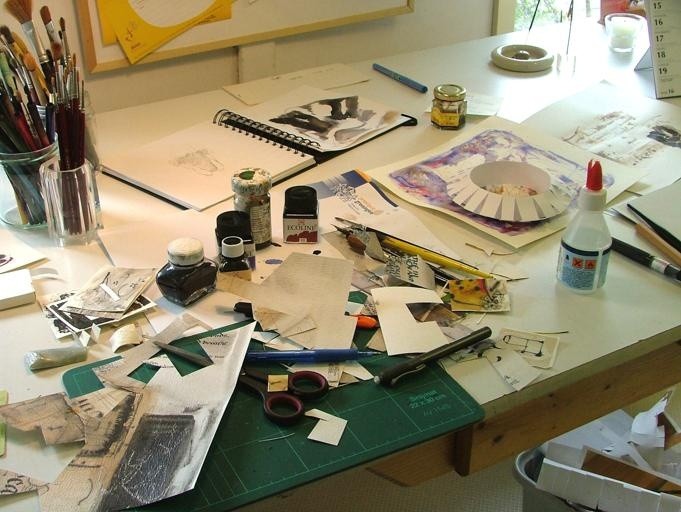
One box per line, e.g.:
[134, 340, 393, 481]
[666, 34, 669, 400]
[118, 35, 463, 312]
[371, 65, 429, 93]
[612, 202, 681, 279]
[375, 325, 493, 389]
[244, 349, 385, 362]
[232, 302, 376, 331]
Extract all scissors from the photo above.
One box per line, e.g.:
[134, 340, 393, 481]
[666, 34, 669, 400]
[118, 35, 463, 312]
[153, 340, 328, 423]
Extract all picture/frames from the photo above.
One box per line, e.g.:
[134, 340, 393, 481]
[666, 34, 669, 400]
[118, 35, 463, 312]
[71, 0, 417, 76]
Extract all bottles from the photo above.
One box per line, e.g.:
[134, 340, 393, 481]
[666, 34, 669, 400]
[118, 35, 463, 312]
[156, 237, 219, 306]
[214, 167, 319, 283]
[555, 160, 613, 295]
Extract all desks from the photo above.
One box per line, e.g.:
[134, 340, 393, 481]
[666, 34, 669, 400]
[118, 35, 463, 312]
[1, 15, 681, 512]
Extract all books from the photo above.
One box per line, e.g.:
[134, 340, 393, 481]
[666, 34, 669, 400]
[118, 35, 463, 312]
[100, 83, 412, 216]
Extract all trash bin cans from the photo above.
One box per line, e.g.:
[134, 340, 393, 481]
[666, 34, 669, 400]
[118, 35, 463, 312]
[515, 445, 595, 510]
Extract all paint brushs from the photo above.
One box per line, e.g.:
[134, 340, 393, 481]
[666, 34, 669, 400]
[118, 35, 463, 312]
[1, 1, 100, 234]
[329, 216, 494, 288]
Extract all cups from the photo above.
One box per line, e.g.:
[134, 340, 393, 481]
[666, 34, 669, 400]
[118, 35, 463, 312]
[604, 13, 646, 53]
